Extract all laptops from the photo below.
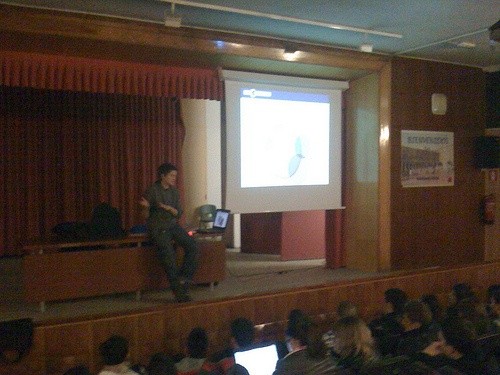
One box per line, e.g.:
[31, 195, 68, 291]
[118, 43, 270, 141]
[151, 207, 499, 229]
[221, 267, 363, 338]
[195, 209, 231, 234]
[232, 341, 280, 375]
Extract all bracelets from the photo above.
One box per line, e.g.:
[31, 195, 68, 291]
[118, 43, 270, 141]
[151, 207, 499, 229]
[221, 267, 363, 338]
[166, 205, 171, 210]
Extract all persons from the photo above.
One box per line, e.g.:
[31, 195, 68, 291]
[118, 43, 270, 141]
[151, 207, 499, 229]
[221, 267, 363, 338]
[63, 281, 500, 375]
[135, 164, 198, 304]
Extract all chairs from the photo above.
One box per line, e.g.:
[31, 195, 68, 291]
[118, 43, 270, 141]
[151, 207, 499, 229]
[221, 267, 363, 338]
[371, 332, 500, 375]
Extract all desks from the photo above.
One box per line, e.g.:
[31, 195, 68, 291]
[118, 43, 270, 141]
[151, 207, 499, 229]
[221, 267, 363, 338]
[21, 230, 226, 313]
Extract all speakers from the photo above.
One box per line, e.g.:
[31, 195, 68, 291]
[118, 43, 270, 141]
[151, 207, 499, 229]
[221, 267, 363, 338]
[485, 71, 500, 128]
[473, 135, 500, 167]
